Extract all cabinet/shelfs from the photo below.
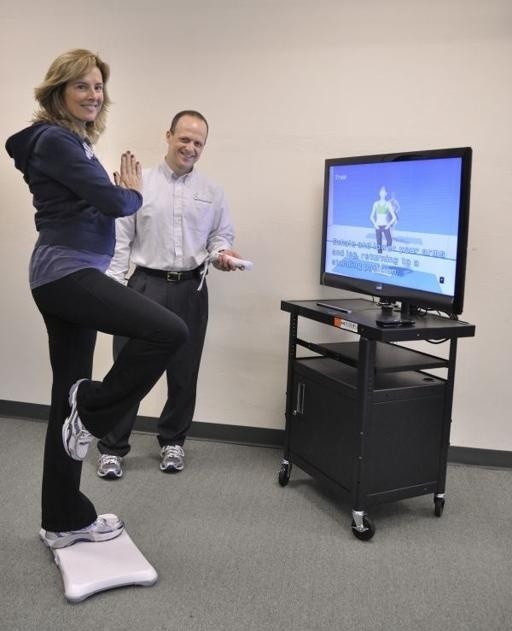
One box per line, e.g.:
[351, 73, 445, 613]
[278, 299, 475, 541]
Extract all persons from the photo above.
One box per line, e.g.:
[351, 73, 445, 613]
[388, 190, 402, 216]
[3, 42, 190, 553]
[369, 185, 397, 255]
[94, 105, 246, 481]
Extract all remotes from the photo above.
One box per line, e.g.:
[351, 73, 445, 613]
[376, 319, 415, 327]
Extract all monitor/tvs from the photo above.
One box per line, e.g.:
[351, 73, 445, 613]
[320, 147, 472, 314]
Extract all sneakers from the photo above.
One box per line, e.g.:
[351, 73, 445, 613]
[159, 444, 184, 472]
[61, 378, 95, 461]
[44, 513, 124, 549]
[98, 454, 123, 478]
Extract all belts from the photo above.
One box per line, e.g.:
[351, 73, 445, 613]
[136, 266, 200, 282]
